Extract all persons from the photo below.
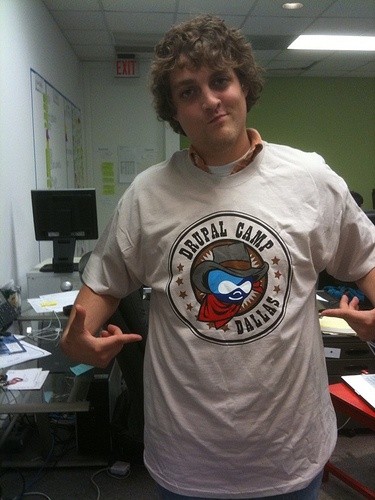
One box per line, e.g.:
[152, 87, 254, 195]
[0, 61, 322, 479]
[58, 15, 375, 500]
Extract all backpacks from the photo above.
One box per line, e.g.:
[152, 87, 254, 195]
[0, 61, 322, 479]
[103, 389, 144, 463]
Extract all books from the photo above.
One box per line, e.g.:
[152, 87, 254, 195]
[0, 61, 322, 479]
[318, 313, 357, 336]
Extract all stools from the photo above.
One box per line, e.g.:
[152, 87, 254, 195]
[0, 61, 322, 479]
[322, 383, 375, 500]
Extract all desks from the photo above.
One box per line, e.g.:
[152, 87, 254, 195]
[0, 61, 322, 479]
[0, 314, 108, 469]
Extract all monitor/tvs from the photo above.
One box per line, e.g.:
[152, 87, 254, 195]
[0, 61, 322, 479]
[31, 189, 99, 273]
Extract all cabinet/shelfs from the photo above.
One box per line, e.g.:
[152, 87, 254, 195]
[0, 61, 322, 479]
[324, 334, 375, 435]
